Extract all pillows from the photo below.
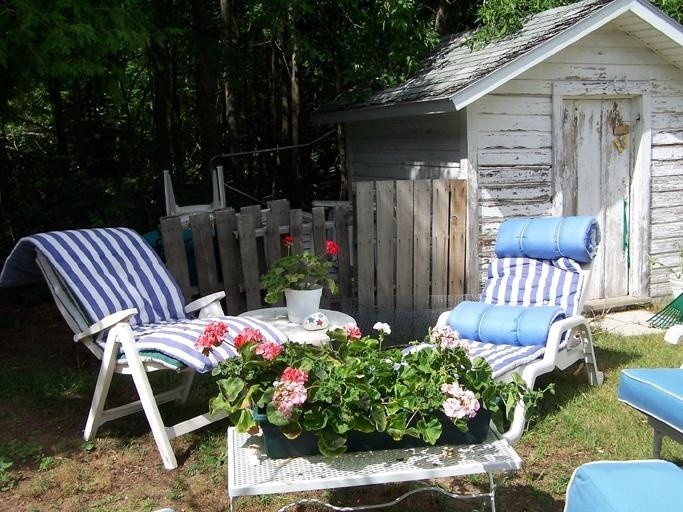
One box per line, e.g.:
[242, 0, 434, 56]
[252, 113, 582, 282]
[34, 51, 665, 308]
[447, 301, 567, 347]
[495, 216, 601, 264]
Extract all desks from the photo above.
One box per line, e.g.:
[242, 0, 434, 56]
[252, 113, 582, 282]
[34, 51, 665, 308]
[227, 426, 522, 512]
[237, 307, 358, 344]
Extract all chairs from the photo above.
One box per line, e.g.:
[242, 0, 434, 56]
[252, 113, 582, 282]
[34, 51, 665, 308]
[1, 225, 289, 471]
[563, 458, 683, 512]
[617, 367, 683, 460]
[380, 216, 600, 448]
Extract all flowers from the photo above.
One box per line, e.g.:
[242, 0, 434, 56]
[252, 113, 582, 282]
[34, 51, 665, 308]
[259, 235, 341, 304]
[194, 320, 555, 459]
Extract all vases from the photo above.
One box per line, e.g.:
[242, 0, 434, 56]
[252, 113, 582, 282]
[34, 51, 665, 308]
[252, 403, 495, 459]
[285, 282, 323, 323]
